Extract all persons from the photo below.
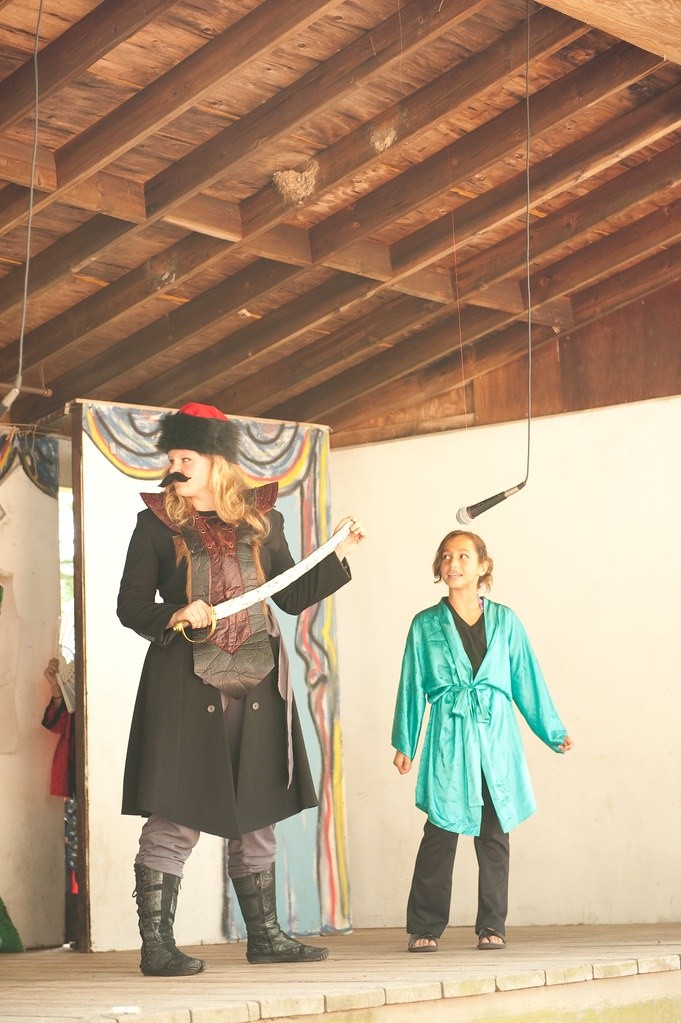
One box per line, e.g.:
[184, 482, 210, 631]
[391, 530, 573, 952]
[116, 402, 366, 976]
[41, 658, 79, 948]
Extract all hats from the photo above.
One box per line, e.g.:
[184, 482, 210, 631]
[154, 402, 240, 464]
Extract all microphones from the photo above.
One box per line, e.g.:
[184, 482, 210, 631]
[456, 482, 525, 526]
[0, 388, 20, 419]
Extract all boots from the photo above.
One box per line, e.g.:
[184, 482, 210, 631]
[131, 863, 207, 976]
[232, 862, 329, 964]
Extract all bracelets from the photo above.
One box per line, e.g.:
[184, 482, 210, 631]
[53, 695, 62, 699]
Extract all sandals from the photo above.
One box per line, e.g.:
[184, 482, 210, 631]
[477, 928, 507, 950]
[408, 931, 439, 952]
